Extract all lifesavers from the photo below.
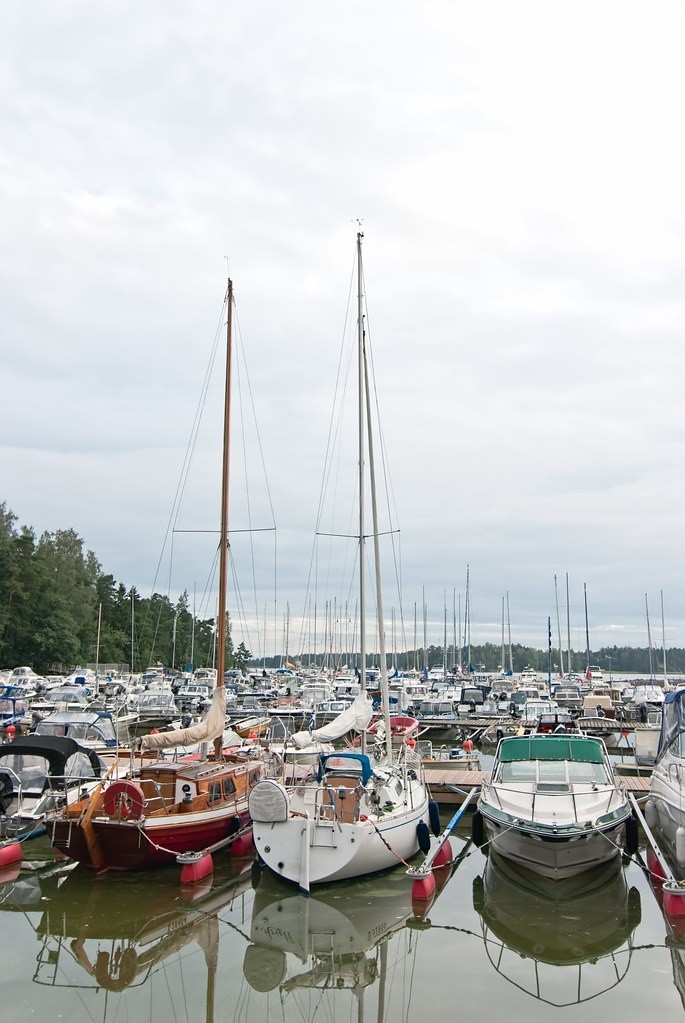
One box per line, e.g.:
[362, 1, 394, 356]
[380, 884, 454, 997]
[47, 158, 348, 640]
[95, 948, 137, 993]
[103, 779, 145, 820]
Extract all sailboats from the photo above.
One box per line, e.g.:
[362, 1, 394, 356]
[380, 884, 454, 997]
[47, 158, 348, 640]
[0, 561, 685, 777]
[247, 847, 433, 1023]
[30, 845, 253, 1023]
[248, 217, 435, 893]
[36, 274, 270, 875]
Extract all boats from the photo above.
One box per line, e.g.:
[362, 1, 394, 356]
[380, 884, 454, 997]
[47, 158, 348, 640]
[643, 681, 685, 874]
[479, 841, 646, 1010]
[1, 733, 137, 851]
[475, 733, 633, 883]
[0, 834, 81, 913]
